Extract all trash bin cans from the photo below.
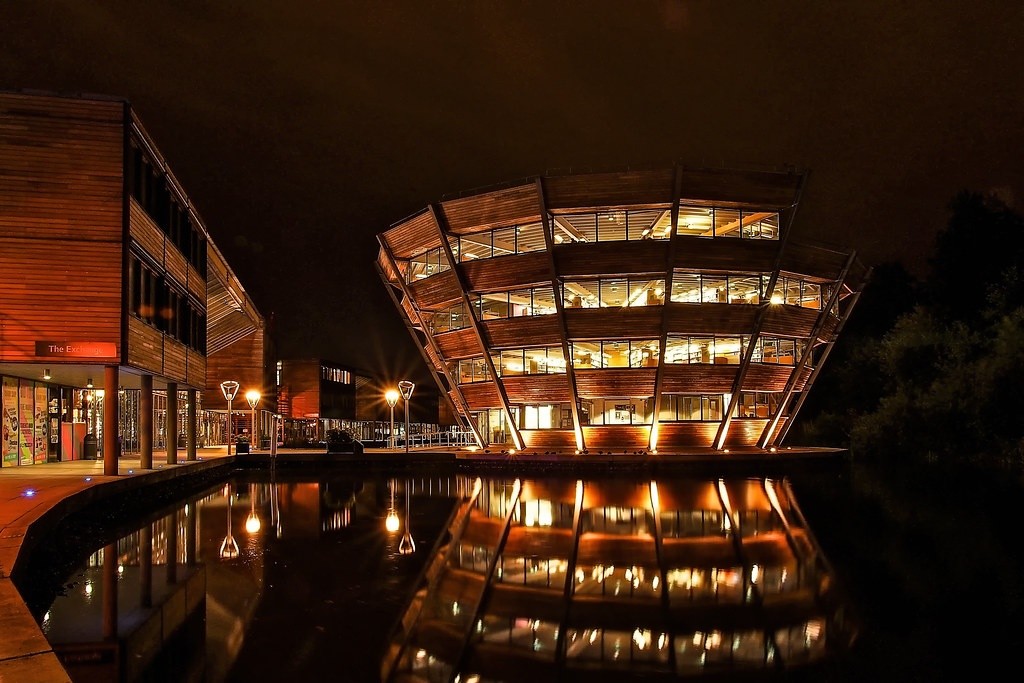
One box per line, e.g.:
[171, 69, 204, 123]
[178, 434, 187, 447]
[84, 434, 97, 459]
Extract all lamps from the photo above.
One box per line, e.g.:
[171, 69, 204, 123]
[426, 266, 434, 274]
[611, 281, 620, 305]
[697, 276, 702, 281]
[709, 209, 714, 216]
[87, 378, 94, 388]
[476, 300, 480, 307]
[451, 247, 457, 254]
[451, 314, 457, 321]
[515, 227, 521, 235]
[120, 385, 124, 394]
[614, 343, 619, 349]
[608, 214, 614, 221]
[43, 368, 51, 380]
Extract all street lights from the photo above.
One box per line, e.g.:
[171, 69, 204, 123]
[386, 477, 399, 531]
[246, 389, 262, 450]
[219, 482, 240, 559]
[385, 390, 400, 449]
[219, 380, 240, 455]
[398, 479, 416, 555]
[398, 379, 415, 452]
[245, 483, 261, 534]
